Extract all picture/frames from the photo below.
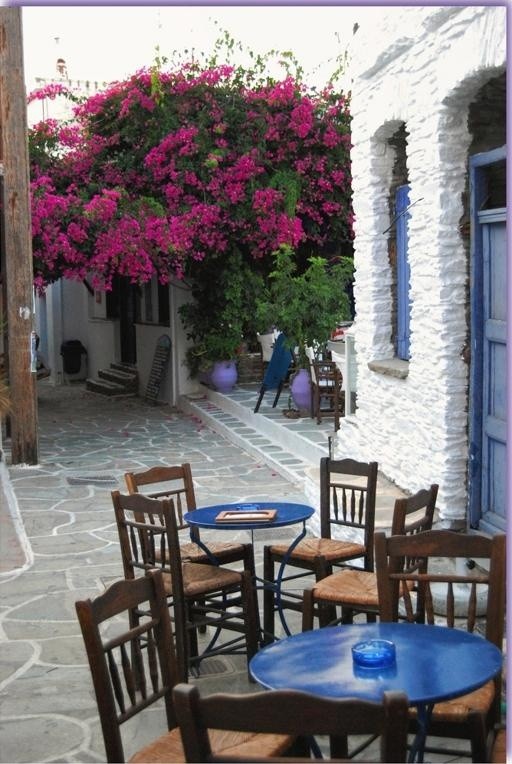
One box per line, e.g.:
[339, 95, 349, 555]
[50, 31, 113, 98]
[215, 509, 277, 523]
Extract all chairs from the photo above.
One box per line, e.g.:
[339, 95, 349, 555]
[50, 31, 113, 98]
[75, 567, 311, 763]
[124, 462, 262, 643]
[263, 456, 378, 645]
[374, 529, 507, 764]
[111, 489, 260, 684]
[302, 483, 438, 634]
[172, 683, 409, 764]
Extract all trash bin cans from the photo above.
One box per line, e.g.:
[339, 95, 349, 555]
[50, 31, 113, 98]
[63, 340, 83, 375]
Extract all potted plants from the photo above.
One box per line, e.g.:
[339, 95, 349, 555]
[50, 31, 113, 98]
[179, 266, 246, 395]
[252, 240, 355, 416]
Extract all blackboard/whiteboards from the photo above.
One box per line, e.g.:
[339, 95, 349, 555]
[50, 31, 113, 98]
[145, 334, 172, 400]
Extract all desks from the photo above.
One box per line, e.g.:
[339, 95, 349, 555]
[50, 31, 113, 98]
[184, 502, 315, 654]
[248, 622, 503, 764]
